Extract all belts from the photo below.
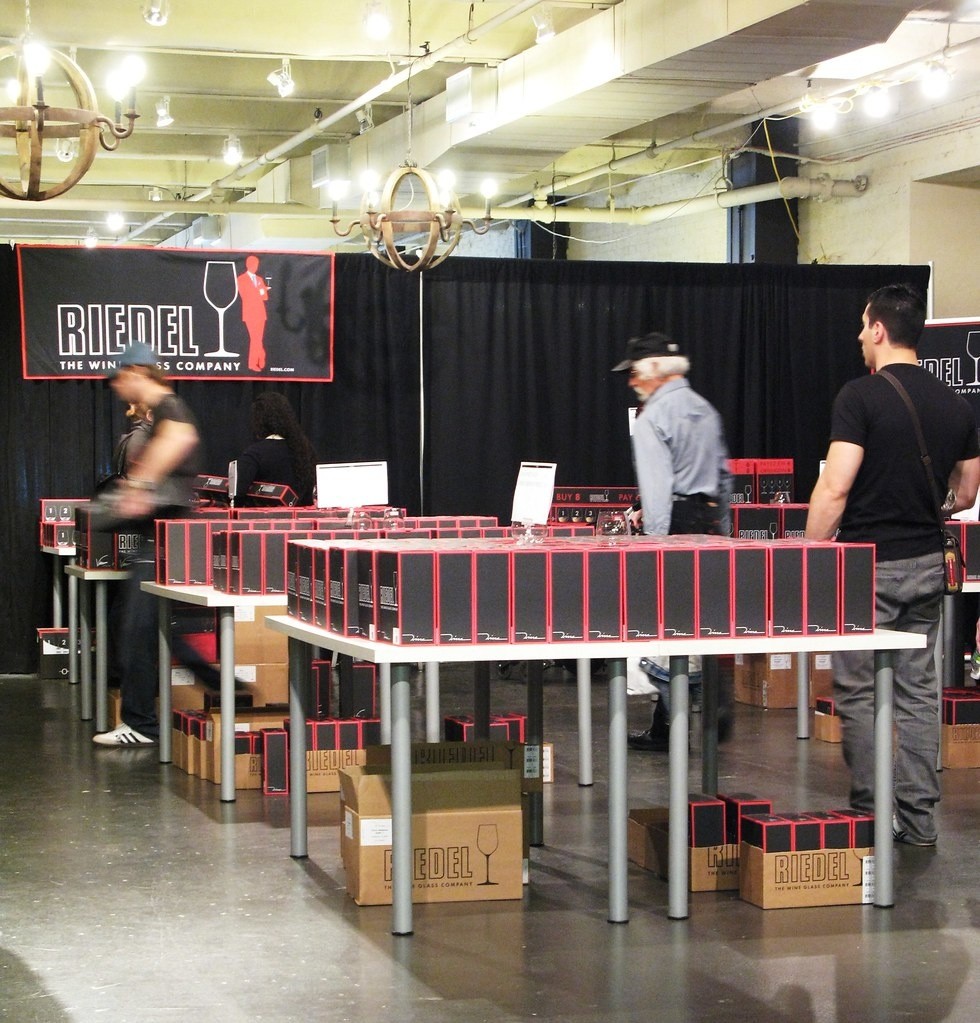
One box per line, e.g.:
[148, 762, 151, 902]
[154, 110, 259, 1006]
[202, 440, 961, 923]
[672, 494, 689, 501]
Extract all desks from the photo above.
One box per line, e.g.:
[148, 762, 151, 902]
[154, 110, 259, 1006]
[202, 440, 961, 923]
[62, 563, 133, 737]
[41, 545, 78, 632]
[137, 581, 287, 805]
[263, 616, 929, 938]
[935, 576, 980, 775]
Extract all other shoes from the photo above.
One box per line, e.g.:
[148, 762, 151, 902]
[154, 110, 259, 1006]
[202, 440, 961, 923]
[628, 729, 669, 751]
[890, 814, 936, 846]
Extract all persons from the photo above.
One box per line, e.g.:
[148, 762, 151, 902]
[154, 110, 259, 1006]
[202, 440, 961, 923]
[610, 334, 738, 752]
[804, 285, 980, 846]
[84, 341, 319, 748]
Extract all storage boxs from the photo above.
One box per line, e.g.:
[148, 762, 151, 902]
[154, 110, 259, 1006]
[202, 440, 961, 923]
[38, 460, 980, 911]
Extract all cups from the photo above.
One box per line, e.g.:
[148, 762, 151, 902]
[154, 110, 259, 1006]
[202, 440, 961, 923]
[383, 507, 406, 530]
[531, 522, 549, 544]
[511, 521, 530, 544]
[596, 511, 632, 547]
[351, 511, 374, 530]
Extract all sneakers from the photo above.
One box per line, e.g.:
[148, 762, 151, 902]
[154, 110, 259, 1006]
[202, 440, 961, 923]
[92, 722, 158, 747]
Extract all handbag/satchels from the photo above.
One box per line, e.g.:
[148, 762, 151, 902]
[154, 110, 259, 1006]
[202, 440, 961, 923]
[87, 437, 128, 505]
[941, 530, 967, 595]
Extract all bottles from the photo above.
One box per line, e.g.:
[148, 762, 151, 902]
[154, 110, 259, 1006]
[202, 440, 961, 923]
[969, 647, 980, 680]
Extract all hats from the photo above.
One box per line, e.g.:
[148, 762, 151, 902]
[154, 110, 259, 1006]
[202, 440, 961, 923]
[610, 331, 680, 372]
[103, 340, 156, 380]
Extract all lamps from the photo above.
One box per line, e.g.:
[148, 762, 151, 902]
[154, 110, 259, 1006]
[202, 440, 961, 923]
[148, 188, 163, 202]
[0, 0, 146, 202]
[354, 104, 375, 135]
[530, 9, 555, 44]
[265, 57, 296, 97]
[223, 132, 243, 163]
[156, 96, 176, 128]
[143, 0, 171, 27]
